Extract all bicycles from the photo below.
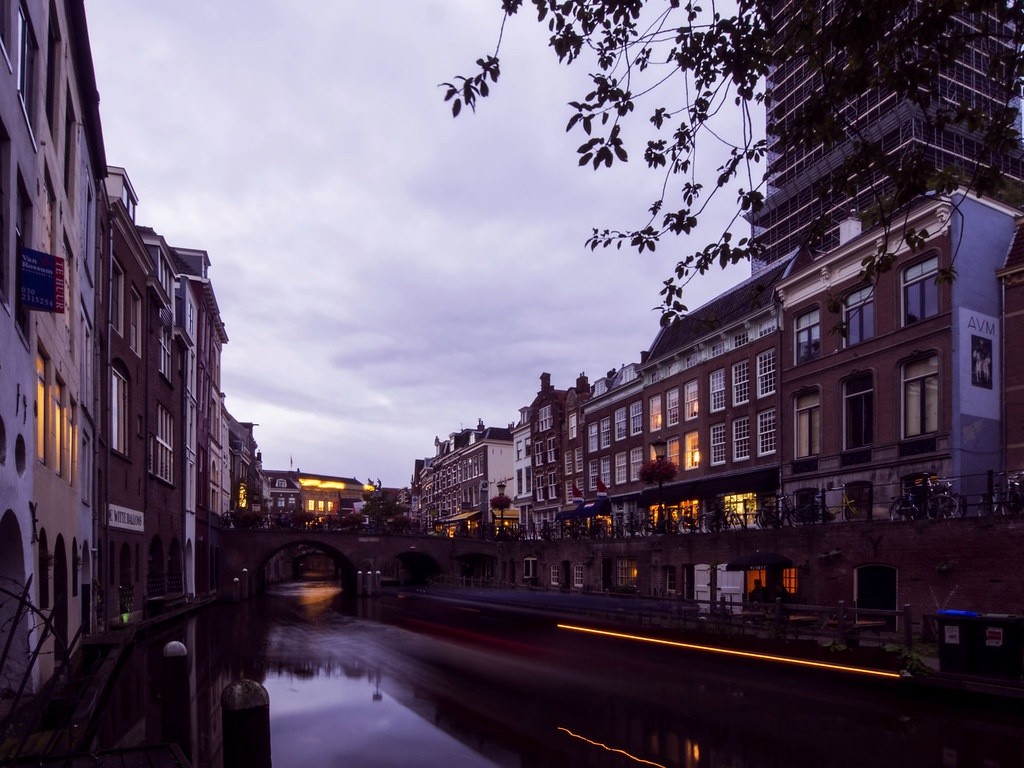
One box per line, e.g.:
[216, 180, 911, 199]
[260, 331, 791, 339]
[888, 471, 960, 521]
[976, 470, 1024, 518]
[540, 503, 748, 542]
[753, 482, 870, 530]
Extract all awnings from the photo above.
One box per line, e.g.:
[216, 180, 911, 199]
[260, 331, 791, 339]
[555, 499, 612, 521]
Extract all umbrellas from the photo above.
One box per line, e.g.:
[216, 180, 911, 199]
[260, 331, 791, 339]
[726, 549, 793, 601]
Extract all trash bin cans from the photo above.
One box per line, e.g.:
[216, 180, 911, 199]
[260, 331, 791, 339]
[932, 608, 983, 676]
[975, 613, 1024, 679]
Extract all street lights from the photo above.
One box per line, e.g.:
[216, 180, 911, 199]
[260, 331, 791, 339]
[651, 438, 666, 534]
[496, 483, 506, 541]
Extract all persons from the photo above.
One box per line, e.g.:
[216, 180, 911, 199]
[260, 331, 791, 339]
[752, 578, 791, 601]
